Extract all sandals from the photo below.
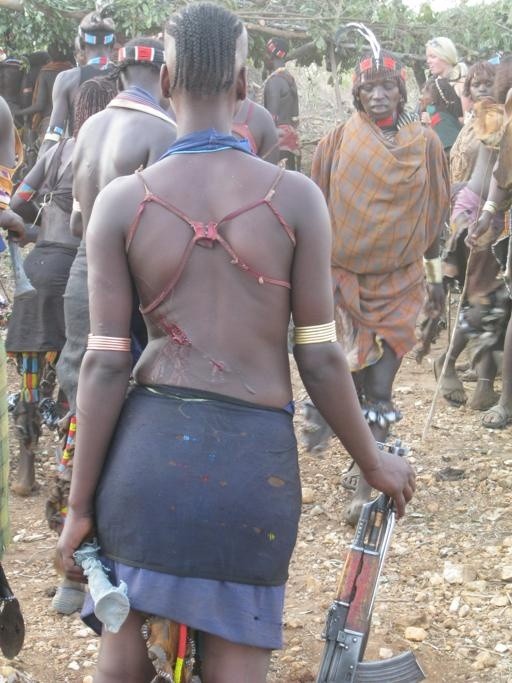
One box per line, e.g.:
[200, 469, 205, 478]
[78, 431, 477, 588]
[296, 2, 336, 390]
[340, 459, 365, 525]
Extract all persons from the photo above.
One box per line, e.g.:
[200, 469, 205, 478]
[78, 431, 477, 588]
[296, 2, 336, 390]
[1, 3, 510, 683]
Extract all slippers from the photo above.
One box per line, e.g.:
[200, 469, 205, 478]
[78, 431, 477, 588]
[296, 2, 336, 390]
[434, 356, 477, 409]
[478, 401, 512, 429]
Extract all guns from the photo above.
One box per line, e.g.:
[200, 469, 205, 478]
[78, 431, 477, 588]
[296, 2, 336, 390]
[317, 436, 423, 682]
